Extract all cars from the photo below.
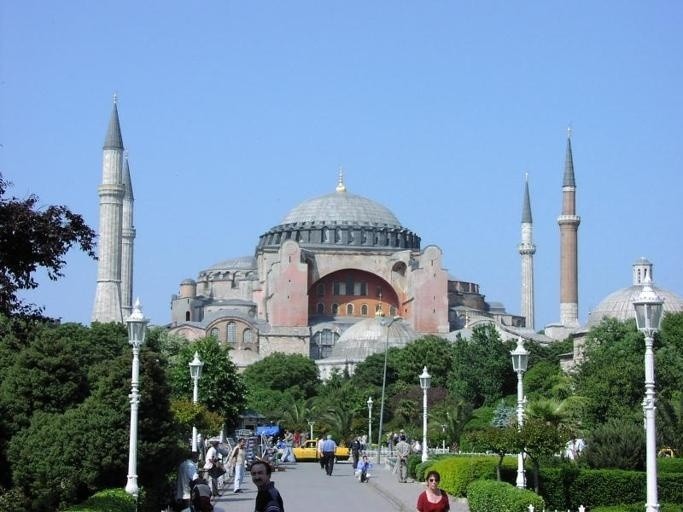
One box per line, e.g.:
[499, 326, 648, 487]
[274, 438, 351, 465]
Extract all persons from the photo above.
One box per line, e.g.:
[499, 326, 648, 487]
[416, 470, 450, 511]
[262, 429, 421, 483]
[175, 437, 246, 512]
[249, 461, 284, 511]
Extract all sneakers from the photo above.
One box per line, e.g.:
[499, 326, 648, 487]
[398, 479, 408, 483]
[234, 488, 243, 493]
[209, 496, 216, 502]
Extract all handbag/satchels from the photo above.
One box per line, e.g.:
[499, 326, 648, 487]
[226, 451, 237, 470]
[209, 466, 227, 480]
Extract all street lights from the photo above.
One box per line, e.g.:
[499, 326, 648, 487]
[507, 332, 535, 489]
[629, 267, 669, 512]
[186, 350, 207, 463]
[439, 424, 447, 454]
[307, 421, 315, 447]
[375, 314, 405, 466]
[365, 395, 374, 447]
[121, 294, 153, 499]
[417, 364, 433, 462]
